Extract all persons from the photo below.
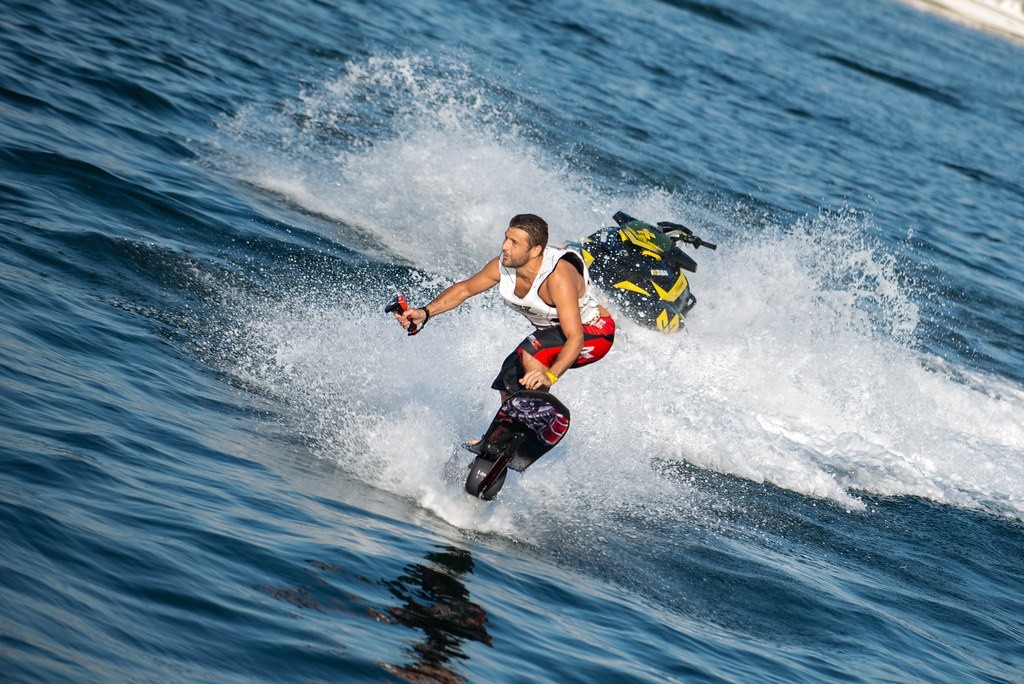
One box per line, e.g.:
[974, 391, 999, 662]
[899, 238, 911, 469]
[395, 214, 615, 445]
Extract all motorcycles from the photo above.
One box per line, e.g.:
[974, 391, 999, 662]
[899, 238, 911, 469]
[561, 210, 718, 340]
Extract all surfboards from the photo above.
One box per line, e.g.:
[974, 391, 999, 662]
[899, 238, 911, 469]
[460, 390, 570, 502]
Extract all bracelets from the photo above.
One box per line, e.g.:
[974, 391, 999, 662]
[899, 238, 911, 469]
[419, 306, 429, 324]
[547, 372, 557, 384]
